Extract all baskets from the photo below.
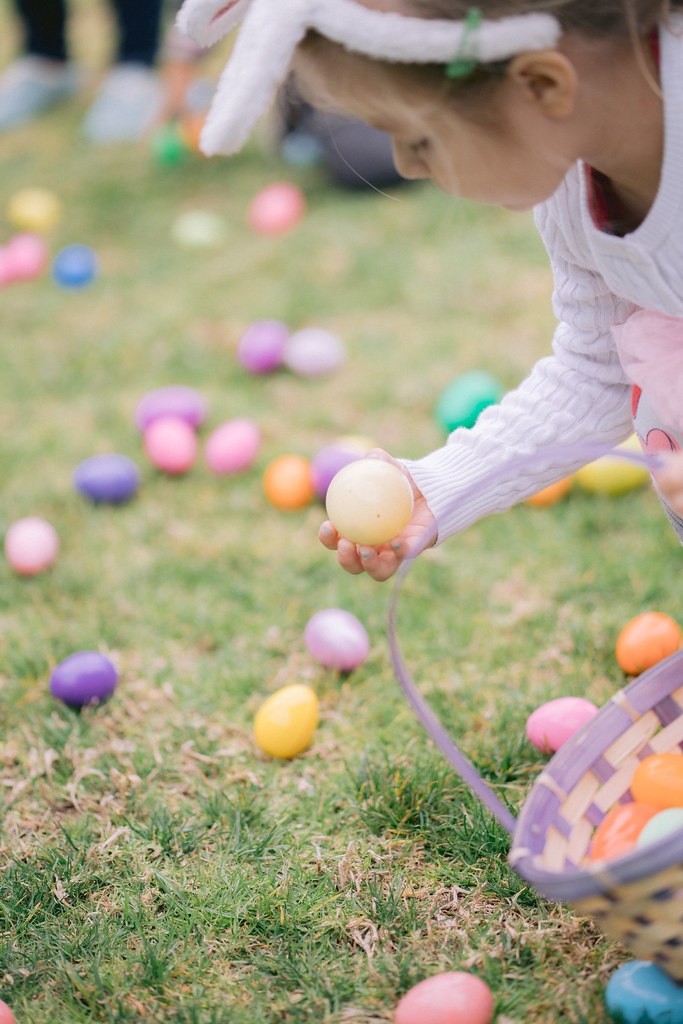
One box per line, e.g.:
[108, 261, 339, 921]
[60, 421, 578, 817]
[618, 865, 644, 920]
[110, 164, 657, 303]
[388, 445, 682, 993]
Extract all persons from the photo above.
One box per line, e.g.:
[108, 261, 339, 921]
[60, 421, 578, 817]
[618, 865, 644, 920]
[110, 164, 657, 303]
[172, 1, 683, 584]
[145, 30, 418, 192]
[0, 0, 166, 143]
